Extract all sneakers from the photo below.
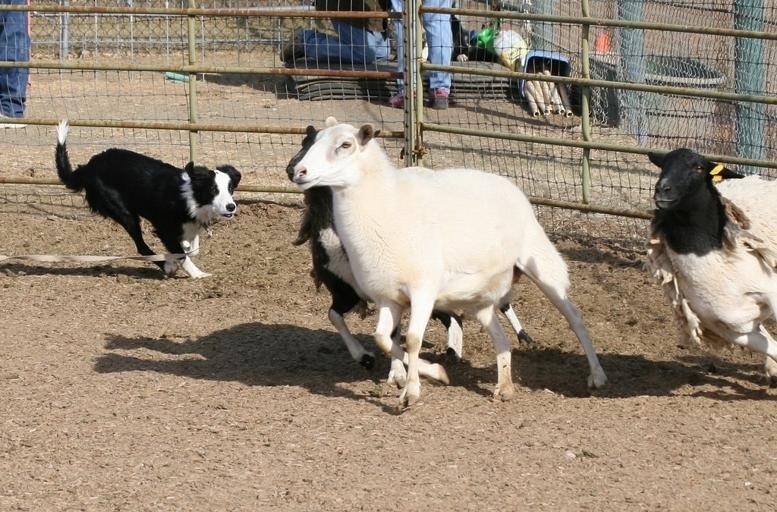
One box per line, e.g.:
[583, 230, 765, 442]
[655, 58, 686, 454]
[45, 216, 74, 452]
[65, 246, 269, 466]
[433, 89, 450, 109]
[389, 94, 411, 109]
[279, 26, 307, 64]
[0, 112, 28, 129]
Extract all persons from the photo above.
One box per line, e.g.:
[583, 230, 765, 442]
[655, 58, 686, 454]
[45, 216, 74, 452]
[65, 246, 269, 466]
[388, 0, 456, 109]
[449, 16, 469, 64]
[0, 0, 31, 129]
[280, 1, 392, 66]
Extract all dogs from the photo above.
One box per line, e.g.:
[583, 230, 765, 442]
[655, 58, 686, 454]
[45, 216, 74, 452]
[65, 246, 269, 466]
[55, 116, 242, 280]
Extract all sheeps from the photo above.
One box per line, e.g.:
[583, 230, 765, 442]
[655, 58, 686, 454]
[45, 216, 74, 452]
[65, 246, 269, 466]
[284, 115, 609, 408]
[646, 148, 777, 390]
